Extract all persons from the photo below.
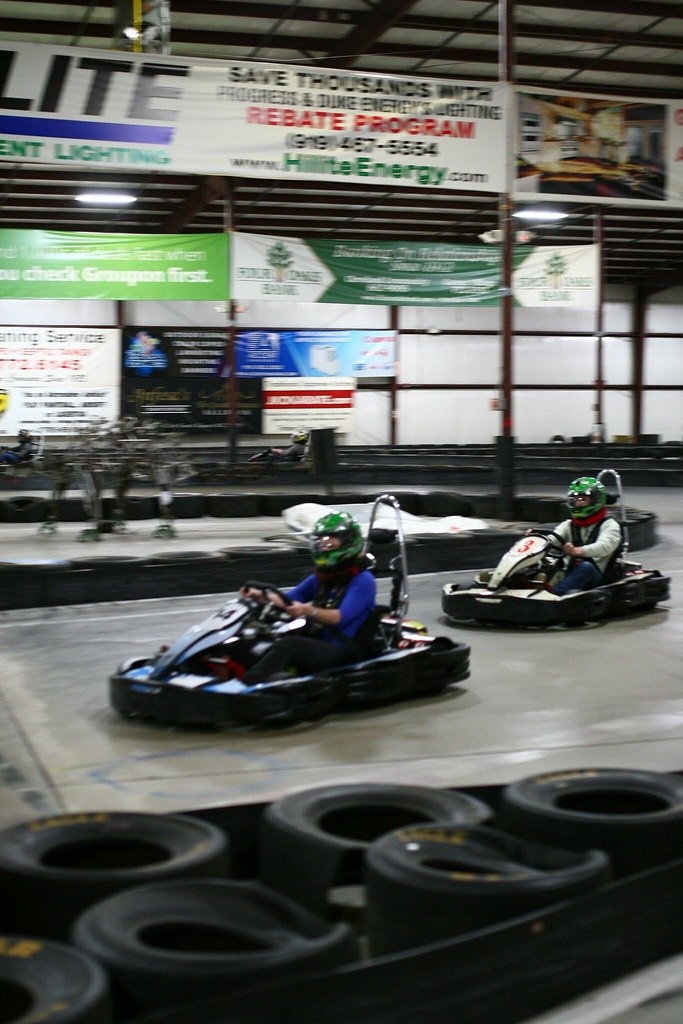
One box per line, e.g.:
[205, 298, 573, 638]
[0, 429, 32, 462]
[158, 510, 377, 686]
[249, 427, 310, 460]
[480, 476, 623, 596]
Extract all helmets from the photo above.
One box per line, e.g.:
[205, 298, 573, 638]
[311, 512, 364, 573]
[567, 476, 606, 519]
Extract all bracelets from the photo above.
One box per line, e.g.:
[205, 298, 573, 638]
[312, 609, 317, 616]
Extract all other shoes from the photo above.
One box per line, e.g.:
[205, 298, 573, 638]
[215, 654, 246, 681]
[535, 573, 553, 593]
[479, 571, 491, 582]
